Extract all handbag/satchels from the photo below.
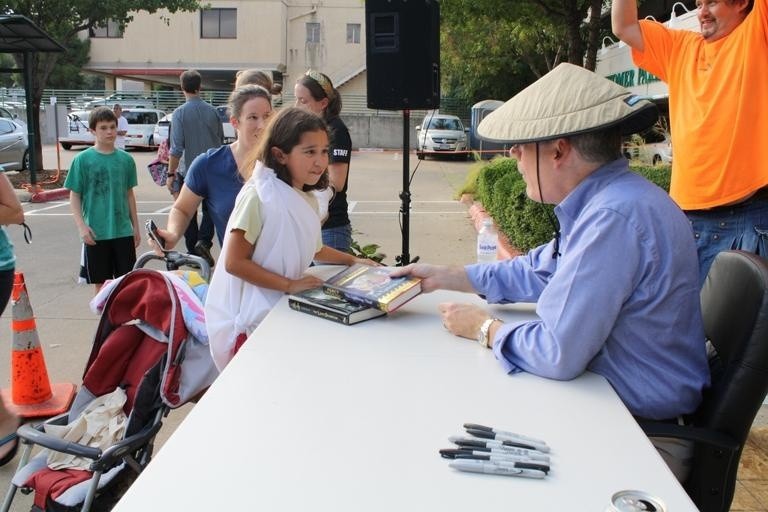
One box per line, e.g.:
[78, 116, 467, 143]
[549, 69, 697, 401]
[148, 160, 168, 187]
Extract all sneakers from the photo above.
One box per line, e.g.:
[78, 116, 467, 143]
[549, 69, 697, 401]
[194, 240, 215, 268]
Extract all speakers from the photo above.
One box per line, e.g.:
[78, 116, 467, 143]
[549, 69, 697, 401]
[364, 0, 442, 111]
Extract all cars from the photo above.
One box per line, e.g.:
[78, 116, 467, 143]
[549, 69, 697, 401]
[0, 115, 31, 173]
[413, 113, 471, 160]
[57, 102, 240, 152]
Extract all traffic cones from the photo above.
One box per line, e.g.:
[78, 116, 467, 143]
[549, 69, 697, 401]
[0, 270, 78, 422]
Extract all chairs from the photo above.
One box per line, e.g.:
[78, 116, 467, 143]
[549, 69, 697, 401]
[637, 249, 768, 512]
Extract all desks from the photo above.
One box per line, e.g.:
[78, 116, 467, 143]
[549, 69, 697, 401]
[109, 265, 701, 512]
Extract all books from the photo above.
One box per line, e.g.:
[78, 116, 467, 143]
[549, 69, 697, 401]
[289, 263, 423, 325]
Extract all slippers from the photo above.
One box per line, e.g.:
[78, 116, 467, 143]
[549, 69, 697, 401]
[0, 418, 25, 467]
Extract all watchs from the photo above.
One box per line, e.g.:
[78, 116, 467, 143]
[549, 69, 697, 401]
[167, 171, 174, 177]
[479, 318, 504, 349]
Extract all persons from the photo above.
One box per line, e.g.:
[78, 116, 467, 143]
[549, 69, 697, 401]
[611, 0, 768, 292]
[389, 62, 712, 487]
[113, 104, 128, 151]
[167, 70, 225, 267]
[0, 165, 25, 461]
[270, 83, 283, 111]
[64, 106, 141, 296]
[235, 69, 273, 92]
[204, 106, 385, 374]
[294, 73, 353, 265]
[147, 84, 273, 258]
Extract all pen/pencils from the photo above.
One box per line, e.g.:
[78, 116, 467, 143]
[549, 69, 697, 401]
[438, 423, 551, 478]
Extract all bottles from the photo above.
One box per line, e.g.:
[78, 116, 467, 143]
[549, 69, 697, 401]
[477, 220, 498, 264]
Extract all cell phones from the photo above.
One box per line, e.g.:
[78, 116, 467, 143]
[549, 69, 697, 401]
[144, 219, 167, 251]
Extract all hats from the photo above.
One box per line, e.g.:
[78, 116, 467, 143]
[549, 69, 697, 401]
[474, 62, 658, 145]
